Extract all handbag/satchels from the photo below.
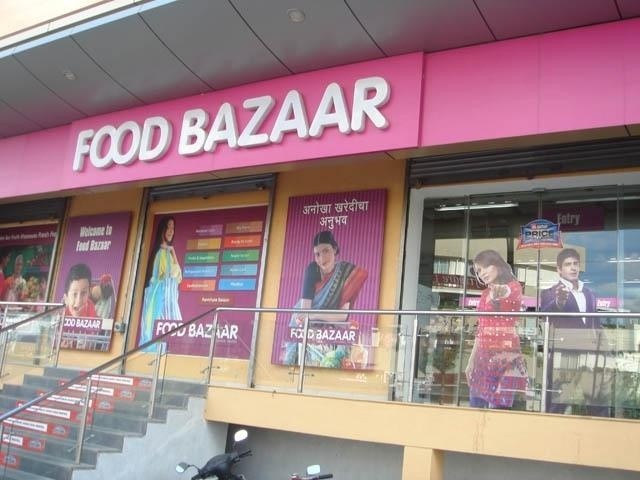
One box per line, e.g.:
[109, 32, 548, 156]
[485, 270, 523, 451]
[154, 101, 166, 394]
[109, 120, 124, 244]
[143, 246, 182, 338]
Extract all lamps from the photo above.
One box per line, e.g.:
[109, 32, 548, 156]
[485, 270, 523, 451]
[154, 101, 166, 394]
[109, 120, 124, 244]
[553, 195, 640, 205]
[433, 199, 518, 213]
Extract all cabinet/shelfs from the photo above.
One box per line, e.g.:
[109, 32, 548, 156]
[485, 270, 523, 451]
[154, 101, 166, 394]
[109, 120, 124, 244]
[430, 257, 537, 342]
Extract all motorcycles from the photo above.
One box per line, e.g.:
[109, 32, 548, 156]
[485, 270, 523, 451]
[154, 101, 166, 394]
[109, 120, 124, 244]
[176, 428, 333, 480]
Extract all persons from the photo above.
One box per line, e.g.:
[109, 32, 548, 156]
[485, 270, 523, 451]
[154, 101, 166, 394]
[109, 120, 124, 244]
[540, 249, 608, 416]
[0, 245, 17, 305]
[285, 230, 370, 369]
[466, 249, 524, 410]
[53, 263, 116, 351]
[137, 216, 183, 354]
[5, 254, 29, 311]
